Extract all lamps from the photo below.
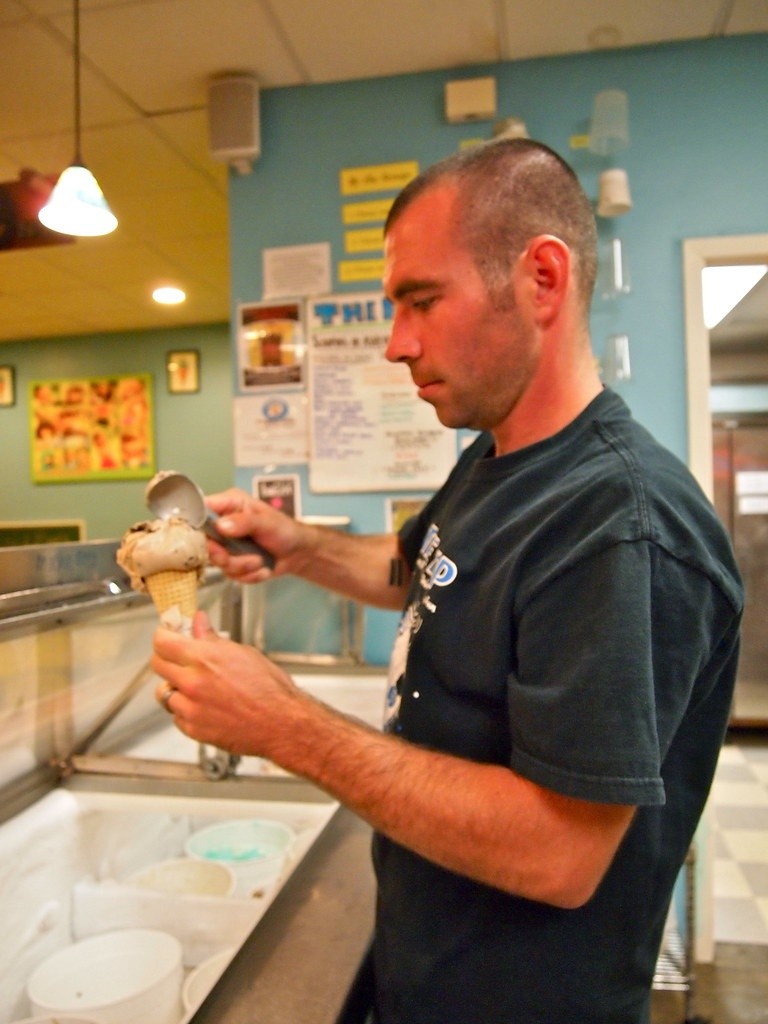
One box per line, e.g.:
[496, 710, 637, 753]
[38, 0, 119, 239]
[593, 168, 632, 219]
[586, 82, 631, 155]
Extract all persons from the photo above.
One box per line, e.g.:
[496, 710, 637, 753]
[149, 137, 744, 1024]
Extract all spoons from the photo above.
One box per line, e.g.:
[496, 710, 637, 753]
[145, 471, 276, 572]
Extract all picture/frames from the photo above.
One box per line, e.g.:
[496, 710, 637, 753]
[26, 370, 157, 486]
[235, 294, 307, 395]
[0, 364, 16, 407]
[165, 349, 201, 395]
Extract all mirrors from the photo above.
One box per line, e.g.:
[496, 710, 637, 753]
[674, 226, 768, 728]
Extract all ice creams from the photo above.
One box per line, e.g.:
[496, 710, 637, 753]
[115, 518, 208, 638]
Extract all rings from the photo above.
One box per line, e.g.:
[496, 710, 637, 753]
[159, 687, 176, 715]
